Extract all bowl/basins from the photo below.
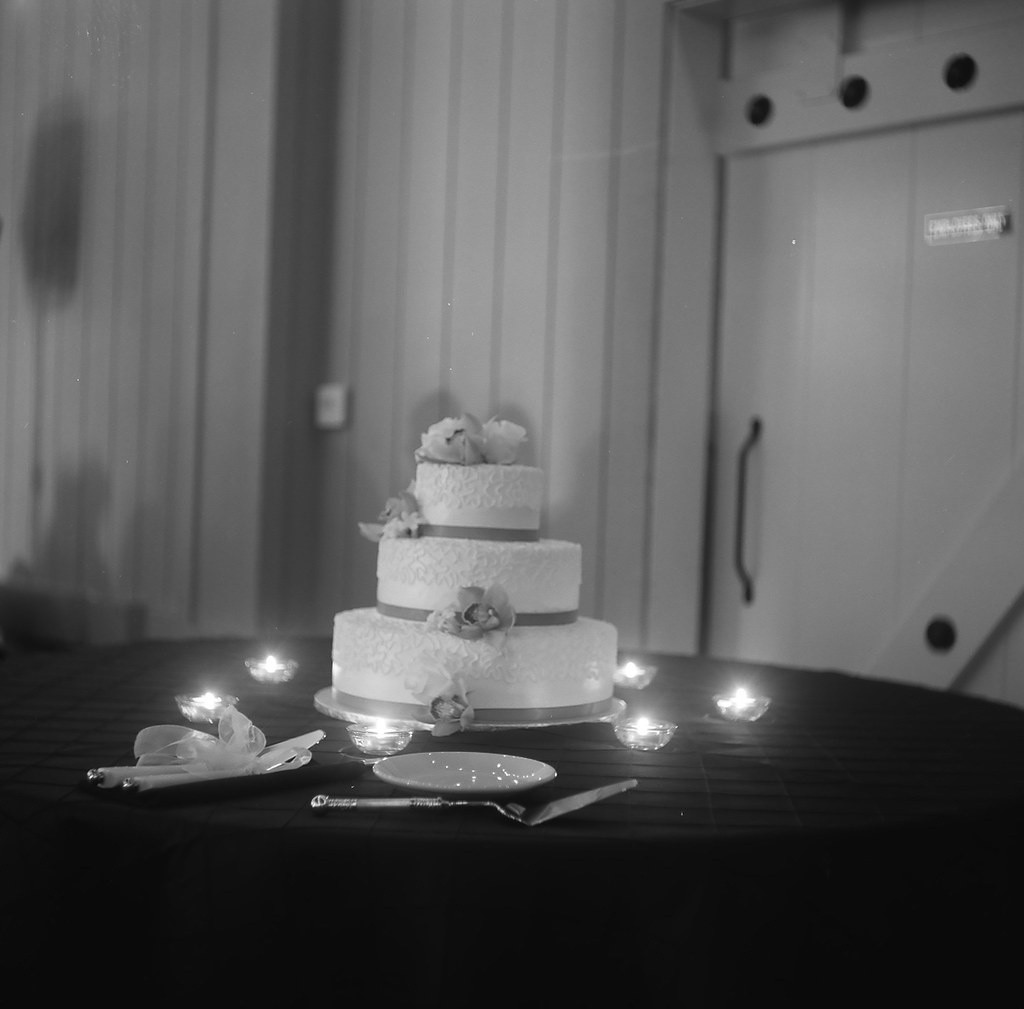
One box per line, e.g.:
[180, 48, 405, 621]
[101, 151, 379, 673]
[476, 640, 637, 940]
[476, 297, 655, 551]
[346, 724, 414, 755]
[711, 694, 771, 723]
[616, 665, 658, 690]
[612, 720, 678, 751]
[244, 659, 297, 683]
[175, 694, 235, 722]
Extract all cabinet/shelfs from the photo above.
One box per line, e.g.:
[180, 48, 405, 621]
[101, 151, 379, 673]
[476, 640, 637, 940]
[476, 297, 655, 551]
[646, 0, 1024, 706]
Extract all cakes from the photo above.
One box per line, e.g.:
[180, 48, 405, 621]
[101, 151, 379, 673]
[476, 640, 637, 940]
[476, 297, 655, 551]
[330, 413, 619, 734]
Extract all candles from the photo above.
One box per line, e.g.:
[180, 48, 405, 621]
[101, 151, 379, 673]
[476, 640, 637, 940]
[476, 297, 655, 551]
[174, 690, 240, 724]
[346, 716, 416, 756]
[615, 660, 657, 690]
[243, 655, 299, 684]
[713, 689, 771, 721]
[614, 716, 678, 750]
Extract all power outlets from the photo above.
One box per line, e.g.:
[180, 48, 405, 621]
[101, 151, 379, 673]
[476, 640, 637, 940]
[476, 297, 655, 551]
[315, 383, 349, 429]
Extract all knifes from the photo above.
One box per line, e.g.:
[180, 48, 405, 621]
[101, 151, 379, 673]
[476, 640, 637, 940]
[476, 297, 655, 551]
[311, 778, 638, 825]
[86, 730, 325, 789]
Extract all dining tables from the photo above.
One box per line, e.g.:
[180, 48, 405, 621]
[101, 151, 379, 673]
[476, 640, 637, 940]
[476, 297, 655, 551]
[0, 640, 1024, 1009]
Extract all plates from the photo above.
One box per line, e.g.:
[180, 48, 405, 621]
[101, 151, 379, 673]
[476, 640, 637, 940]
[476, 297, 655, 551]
[372, 752, 557, 801]
